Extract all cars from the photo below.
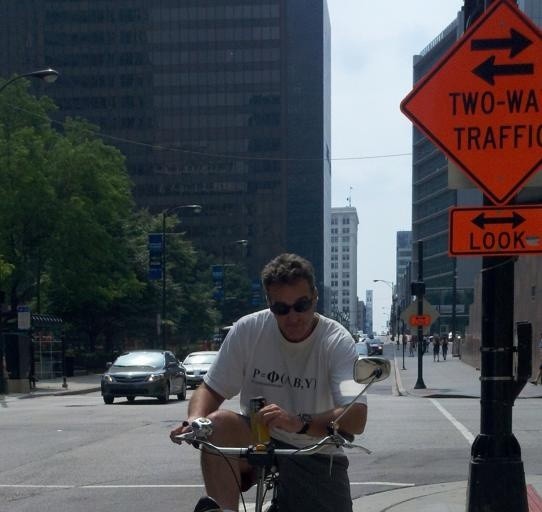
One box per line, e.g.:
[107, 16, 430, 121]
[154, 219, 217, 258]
[423, 335, 435, 342]
[177, 349, 218, 389]
[449, 331, 462, 341]
[97, 347, 189, 404]
[352, 329, 384, 358]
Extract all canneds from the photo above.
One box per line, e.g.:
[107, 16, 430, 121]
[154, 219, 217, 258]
[250, 396, 270, 444]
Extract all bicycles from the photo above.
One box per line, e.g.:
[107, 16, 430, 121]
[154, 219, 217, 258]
[174, 357, 396, 512]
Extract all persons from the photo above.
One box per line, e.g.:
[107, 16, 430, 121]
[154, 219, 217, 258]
[408, 337, 414, 358]
[441, 335, 449, 360]
[171, 250, 369, 512]
[1, 341, 14, 391]
[429, 335, 433, 342]
[432, 336, 441, 363]
[423, 335, 427, 351]
[400, 331, 408, 353]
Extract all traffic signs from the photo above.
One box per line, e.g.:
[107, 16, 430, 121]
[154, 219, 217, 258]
[448, 205, 542, 256]
[399, 0, 542, 208]
[410, 315, 431, 326]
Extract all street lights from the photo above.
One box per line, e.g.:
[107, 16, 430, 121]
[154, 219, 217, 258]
[161, 204, 205, 348]
[372, 277, 395, 342]
[1, 68, 59, 96]
[220, 238, 250, 334]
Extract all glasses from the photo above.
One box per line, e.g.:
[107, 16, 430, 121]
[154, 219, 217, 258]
[265, 295, 313, 315]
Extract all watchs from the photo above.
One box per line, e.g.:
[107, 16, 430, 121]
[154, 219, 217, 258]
[297, 412, 313, 436]
